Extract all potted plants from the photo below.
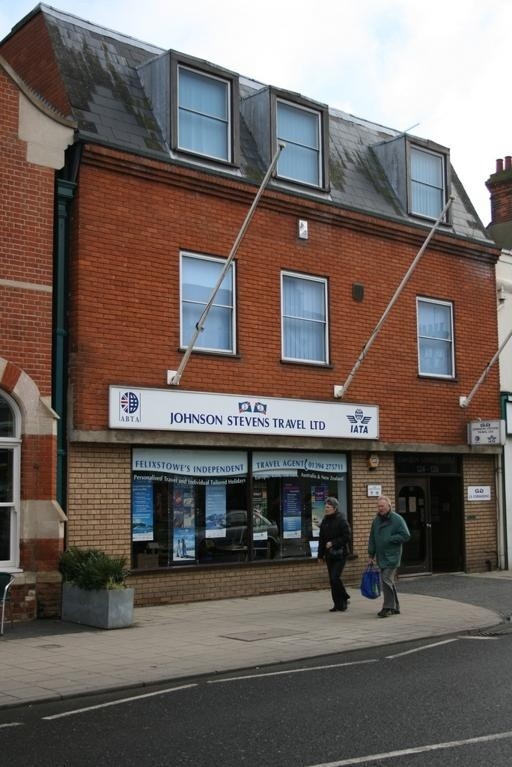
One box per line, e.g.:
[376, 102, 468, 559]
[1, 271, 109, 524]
[56, 546, 136, 629]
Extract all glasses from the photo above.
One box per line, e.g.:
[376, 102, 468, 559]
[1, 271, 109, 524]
[325, 506, 333, 509]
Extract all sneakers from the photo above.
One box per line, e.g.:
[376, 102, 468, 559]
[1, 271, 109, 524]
[329, 593, 350, 612]
[377, 607, 400, 617]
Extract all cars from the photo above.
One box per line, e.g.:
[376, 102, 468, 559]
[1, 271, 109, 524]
[146, 510, 320, 559]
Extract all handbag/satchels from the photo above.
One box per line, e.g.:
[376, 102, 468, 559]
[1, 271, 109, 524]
[360, 568, 382, 599]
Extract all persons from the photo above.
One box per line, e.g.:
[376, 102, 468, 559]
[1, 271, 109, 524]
[239, 503, 272, 562]
[318, 496, 411, 618]
